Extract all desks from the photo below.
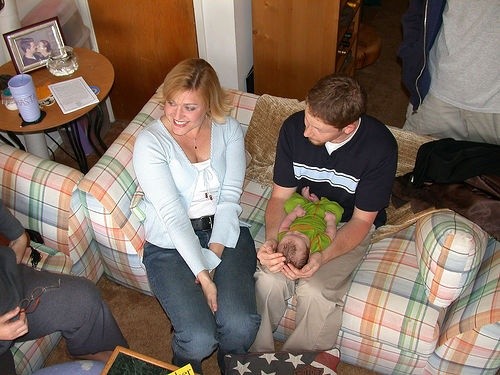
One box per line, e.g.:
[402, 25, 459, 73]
[0, 48, 114, 176]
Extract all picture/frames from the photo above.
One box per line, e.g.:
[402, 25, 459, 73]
[100, 345, 198, 375]
[3, 16, 70, 75]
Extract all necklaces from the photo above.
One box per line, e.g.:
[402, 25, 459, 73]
[185, 136, 199, 149]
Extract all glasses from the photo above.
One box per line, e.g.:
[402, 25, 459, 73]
[19, 278, 61, 313]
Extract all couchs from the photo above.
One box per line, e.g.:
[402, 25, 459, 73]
[0, 141, 105, 375]
[79, 82, 499, 375]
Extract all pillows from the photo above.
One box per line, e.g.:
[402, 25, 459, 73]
[225, 347, 340, 375]
[414, 209, 488, 308]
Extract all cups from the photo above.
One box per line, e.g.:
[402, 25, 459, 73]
[9, 75, 42, 124]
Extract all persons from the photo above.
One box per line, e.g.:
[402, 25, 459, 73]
[0, 200, 130, 375]
[131, 58, 262, 375]
[277, 185, 344, 270]
[248, 73, 399, 354]
[21, 38, 53, 66]
[397, 0, 500, 145]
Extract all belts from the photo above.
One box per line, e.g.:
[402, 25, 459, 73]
[190, 216, 214, 231]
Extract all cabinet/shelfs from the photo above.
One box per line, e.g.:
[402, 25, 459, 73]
[252, 1, 360, 100]
